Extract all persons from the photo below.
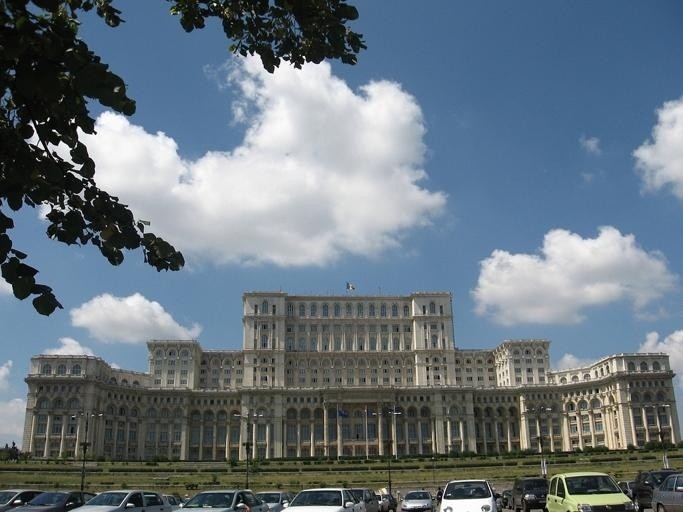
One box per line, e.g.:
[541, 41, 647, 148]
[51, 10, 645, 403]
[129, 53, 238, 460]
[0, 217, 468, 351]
[436, 486, 442, 505]
[184, 493, 190, 502]
[236, 499, 250, 511]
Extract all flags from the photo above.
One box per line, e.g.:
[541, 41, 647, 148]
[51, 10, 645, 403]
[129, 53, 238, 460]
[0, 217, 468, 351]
[346, 282, 355, 290]
[337, 408, 349, 417]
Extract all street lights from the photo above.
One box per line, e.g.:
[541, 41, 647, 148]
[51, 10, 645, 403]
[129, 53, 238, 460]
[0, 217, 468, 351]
[521, 406, 552, 480]
[371, 406, 401, 495]
[233, 412, 264, 490]
[70, 409, 103, 493]
[641, 402, 671, 470]
